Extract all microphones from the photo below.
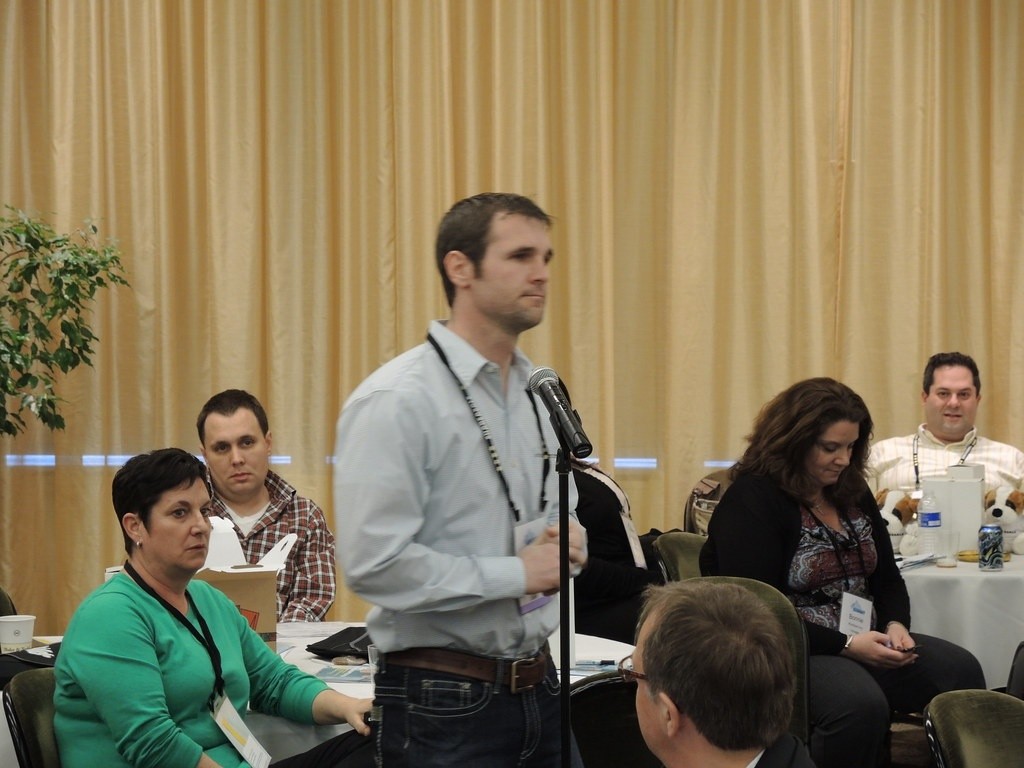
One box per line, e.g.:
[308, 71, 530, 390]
[527, 365, 593, 458]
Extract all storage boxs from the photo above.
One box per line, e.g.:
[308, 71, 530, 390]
[105, 566, 277, 658]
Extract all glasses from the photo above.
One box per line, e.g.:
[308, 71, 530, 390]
[618, 655, 684, 714]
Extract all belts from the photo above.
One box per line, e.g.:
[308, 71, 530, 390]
[377, 639, 551, 694]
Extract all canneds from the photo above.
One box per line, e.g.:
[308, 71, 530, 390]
[977, 524, 1005, 572]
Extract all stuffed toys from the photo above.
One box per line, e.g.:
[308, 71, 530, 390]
[875, 489, 919, 553]
[984, 487, 1024, 554]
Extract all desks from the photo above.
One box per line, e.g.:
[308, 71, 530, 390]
[0, 622, 635, 768]
[890, 554, 1024, 692]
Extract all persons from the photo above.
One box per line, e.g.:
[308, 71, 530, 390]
[633, 582, 816, 768]
[331, 193, 648, 768]
[53, 445, 373, 768]
[196, 389, 337, 622]
[862, 351, 1024, 494]
[699, 377, 986, 768]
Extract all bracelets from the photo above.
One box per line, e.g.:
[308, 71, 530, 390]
[840, 636, 853, 657]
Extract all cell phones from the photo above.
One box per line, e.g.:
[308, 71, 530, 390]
[902, 644, 924, 653]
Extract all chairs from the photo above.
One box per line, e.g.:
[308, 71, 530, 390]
[923, 688, 1024, 768]
[3, 668, 61, 768]
[653, 467, 811, 755]
[0, 587, 17, 616]
